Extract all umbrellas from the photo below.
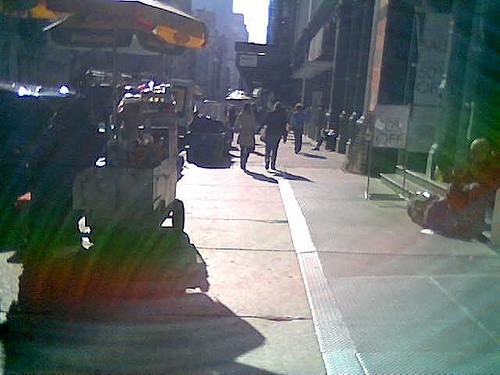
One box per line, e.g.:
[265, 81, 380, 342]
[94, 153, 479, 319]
[0, 0, 208, 114]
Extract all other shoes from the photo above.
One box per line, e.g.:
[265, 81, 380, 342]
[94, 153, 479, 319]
[295, 149, 300, 154]
[240, 164, 243, 169]
[243, 166, 246, 171]
[270, 163, 275, 170]
[265, 160, 269, 169]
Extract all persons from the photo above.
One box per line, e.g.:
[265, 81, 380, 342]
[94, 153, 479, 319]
[233, 102, 256, 169]
[258, 102, 287, 169]
[291, 104, 305, 153]
[229, 101, 291, 134]
